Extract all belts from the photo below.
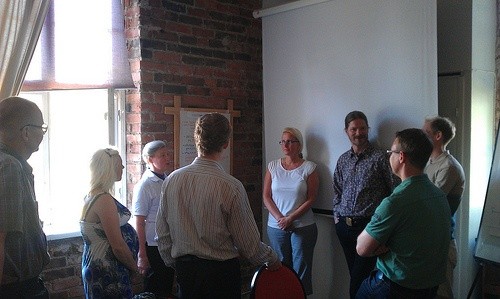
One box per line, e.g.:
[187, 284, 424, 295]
[377, 269, 384, 279]
[176, 256, 199, 262]
[341, 216, 356, 226]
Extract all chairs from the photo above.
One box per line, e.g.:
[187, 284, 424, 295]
[250, 261, 307, 299]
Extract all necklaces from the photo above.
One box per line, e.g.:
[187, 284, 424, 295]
[283, 157, 302, 170]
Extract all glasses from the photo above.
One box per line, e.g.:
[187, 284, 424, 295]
[278, 140, 298, 145]
[383, 148, 400, 157]
[20, 122, 48, 132]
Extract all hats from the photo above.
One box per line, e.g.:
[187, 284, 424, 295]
[345, 110, 367, 125]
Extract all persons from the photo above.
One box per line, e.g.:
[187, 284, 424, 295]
[80, 148, 146, 299]
[132, 140, 175, 299]
[0, 97, 53, 299]
[263, 126, 319, 299]
[332, 111, 400, 299]
[153, 113, 283, 299]
[350, 116, 464, 299]
